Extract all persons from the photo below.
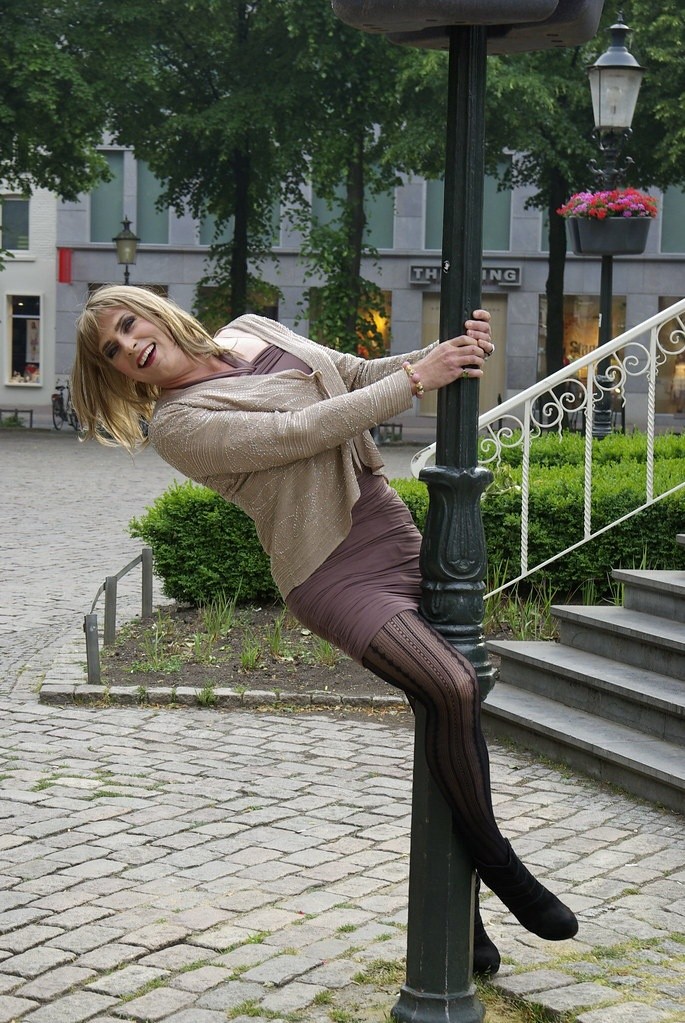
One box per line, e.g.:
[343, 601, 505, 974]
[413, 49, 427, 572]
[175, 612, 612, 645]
[565, 349, 580, 378]
[71, 286, 579, 976]
[673, 358, 685, 412]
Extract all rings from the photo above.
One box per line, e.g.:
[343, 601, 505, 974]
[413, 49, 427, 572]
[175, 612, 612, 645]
[489, 344, 495, 354]
[462, 367, 469, 378]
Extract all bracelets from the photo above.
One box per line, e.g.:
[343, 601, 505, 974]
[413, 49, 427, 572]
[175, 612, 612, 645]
[402, 362, 424, 398]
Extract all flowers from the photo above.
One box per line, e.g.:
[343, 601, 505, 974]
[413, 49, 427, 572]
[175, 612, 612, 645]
[557, 187, 659, 220]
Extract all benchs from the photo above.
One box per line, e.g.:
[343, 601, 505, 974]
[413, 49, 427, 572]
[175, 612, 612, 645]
[0, 404, 33, 428]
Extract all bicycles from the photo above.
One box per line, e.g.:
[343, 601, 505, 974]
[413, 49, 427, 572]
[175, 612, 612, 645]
[51, 378, 82, 431]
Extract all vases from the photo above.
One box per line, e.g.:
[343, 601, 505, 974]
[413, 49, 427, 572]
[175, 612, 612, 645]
[567, 216, 650, 255]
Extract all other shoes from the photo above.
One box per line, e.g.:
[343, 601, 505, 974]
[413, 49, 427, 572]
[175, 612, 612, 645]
[479, 837, 579, 941]
[473, 909, 501, 976]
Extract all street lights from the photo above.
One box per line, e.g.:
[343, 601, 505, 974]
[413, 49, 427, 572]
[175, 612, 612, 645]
[112, 215, 143, 286]
[581, 9, 652, 435]
